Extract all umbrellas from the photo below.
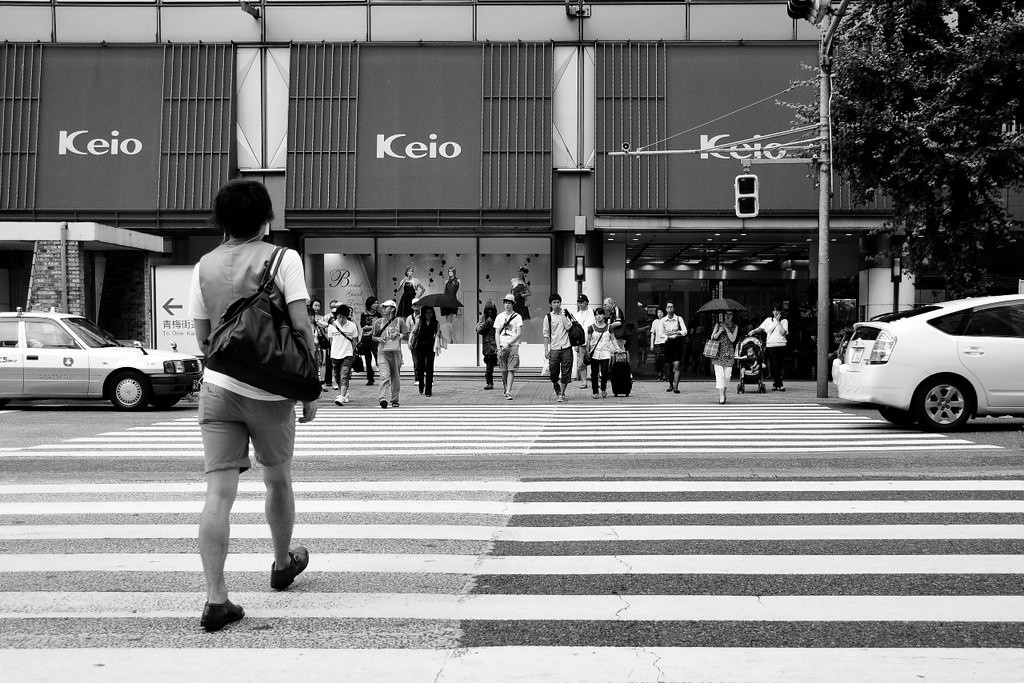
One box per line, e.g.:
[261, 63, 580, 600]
[415, 293, 464, 310]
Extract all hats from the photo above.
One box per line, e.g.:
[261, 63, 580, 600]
[412, 298, 419, 304]
[577, 294, 588, 301]
[502, 294, 517, 304]
[593, 308, 605, 315]
[380, 300, 397, 309]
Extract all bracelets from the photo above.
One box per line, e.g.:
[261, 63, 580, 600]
[508, 343, 510, 347]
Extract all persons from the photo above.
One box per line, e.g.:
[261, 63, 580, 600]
[710, 309, 738, 404]
[635, 311, 653, 365]
[508, 266, 534, 320]
[748, 302, 789, 392]
[732, 344, 762, 379]
[187, 180, 320, 633]
[695, 298, 748, 323]
[475, 299, 499, 390]
[397, 265, 425, 322]
[603, 298, 628, 352]
[306, 296, 441, 407]
[439, 267, 462, 344]
[494, 293, 523, 400]
[660, 299, 688, 393]
[571, 294, 594, 389]
[650, 308, 668, 381]
[587, 307, 622, 399]
[542, 293, 573, 402]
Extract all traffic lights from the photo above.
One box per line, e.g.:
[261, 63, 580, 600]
[732, 172, 761, 219]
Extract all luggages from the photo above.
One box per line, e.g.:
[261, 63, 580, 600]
[611, 352, 633, 397]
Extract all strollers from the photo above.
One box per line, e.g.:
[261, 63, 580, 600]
[734, 335, 768, 394]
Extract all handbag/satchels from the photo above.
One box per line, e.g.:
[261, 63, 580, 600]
[566, 309, 585, 346]
[542, 356, 550, 376]
[313, 320, 330, 349]
[584, 352, 593, 364]
[409, 330, 418, 349]
[315, 345, 323, 368]
[703, 340, 719, 358]
[204, 247, 322, 402]
[353, 354, 364, 372]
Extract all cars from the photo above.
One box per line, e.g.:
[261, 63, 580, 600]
[0, 307, 203, 413]
[832, 294, 1024, 433]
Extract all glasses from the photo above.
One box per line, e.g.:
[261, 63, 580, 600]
[382, 306, 388, 308]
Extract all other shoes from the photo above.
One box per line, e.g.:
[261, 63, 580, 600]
[557, 394, 564, 401]
[719, 397, 726, 404]
[432, 382, 435, 385]
[674, 390, 680, 393]
[580, 385, 587, 389]
[663, 375, 668, 378]
[200, 597, 245, 631]
[593, 393, 599, 398]
[379, 399, 388, 408]
[503, 389, 507, 396]
[269, 545, 309, 590]
[601, 392, 607, 397]
[772, 388, 778, 391]
[414, 381, 419, 385]
[335, 393, 350, 406]
[427, 395, 430, 396]
[484, 385, 493, 389]
[392, 402, 399, 407]
[505, 396, 513, 400]
[333, 383, 338, 390]
[780, 387, 785, 391]
[667, 387, 674, 391]
[553, 383, 561, 395]
[366, 380, 374, 385]
